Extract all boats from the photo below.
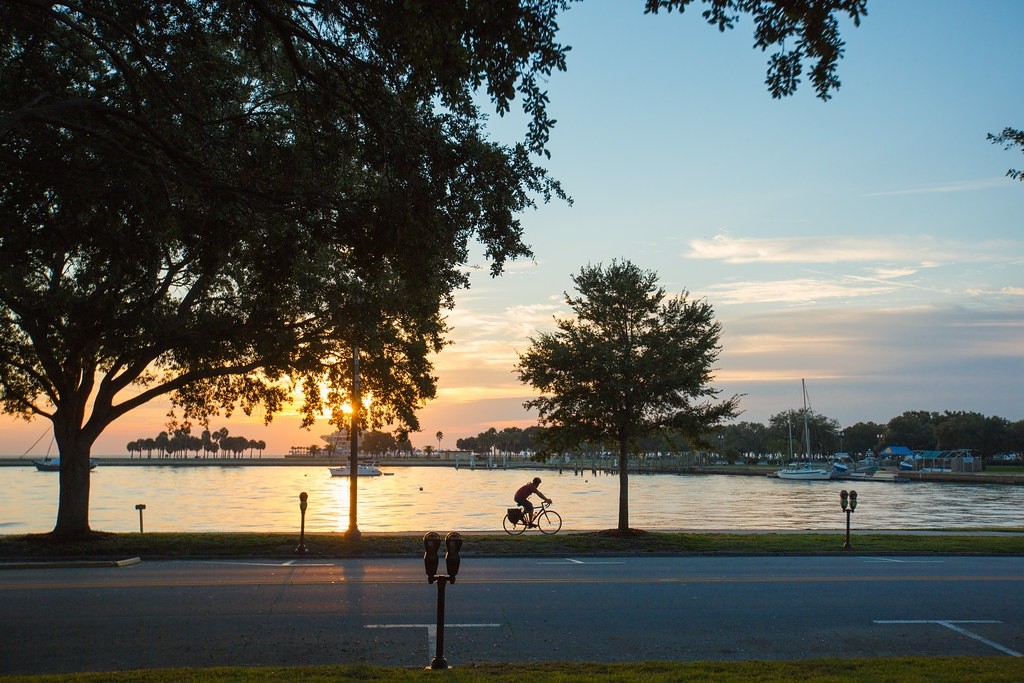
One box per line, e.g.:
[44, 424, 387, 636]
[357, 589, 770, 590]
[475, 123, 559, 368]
[328, 459, 382, 477]
[30, 454, 102, 473]
[766, 471, 778, 478]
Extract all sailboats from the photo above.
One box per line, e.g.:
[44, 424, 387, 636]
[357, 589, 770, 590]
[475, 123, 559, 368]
[777, 379, 833, 479]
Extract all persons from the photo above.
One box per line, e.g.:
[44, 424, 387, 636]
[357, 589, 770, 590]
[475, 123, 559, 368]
[514, 478, 552, 529]
[838, 457, 844, 464]
[796, 463, 800, 470]
[778, 455, 782, 466]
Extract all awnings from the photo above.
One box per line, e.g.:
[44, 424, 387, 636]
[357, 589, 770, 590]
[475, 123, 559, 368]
[879, 446, 916, 468]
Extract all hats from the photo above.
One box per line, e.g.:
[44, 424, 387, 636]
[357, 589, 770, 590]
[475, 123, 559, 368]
[533, 478, 541, 483]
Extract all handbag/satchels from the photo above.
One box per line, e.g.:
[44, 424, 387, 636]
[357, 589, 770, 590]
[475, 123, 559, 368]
[508, 508, 521, 529]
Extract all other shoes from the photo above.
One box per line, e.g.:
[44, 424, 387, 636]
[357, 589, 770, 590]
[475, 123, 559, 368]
[529, 524, 535, 528]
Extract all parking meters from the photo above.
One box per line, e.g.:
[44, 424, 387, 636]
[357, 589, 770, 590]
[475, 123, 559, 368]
[294, 492, 310, 555]
[838, 488, 858, 550]
[421, 530, 463, 671]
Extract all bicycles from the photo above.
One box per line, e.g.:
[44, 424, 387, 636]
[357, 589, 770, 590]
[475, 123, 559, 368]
[502, 499, 562, 536]
